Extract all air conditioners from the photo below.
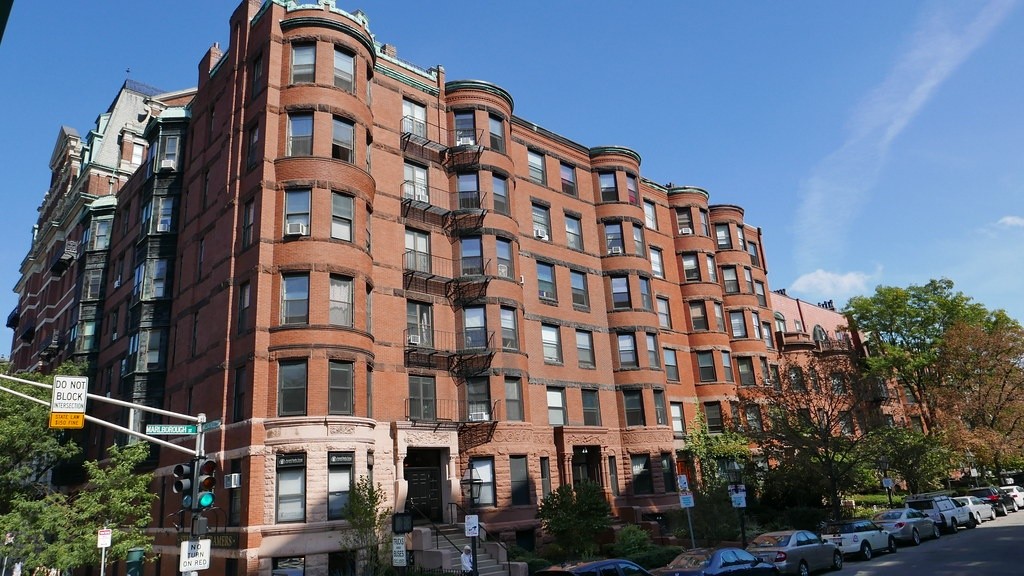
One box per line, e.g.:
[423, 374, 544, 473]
[683, 227, 691, 235]
[408, 335, 420, 345]
[418, 194, 429, 203]
[765, 379, 772, 386]
[114, 281, 119, 289]
[223, 472, 240, 489]
[536, 229, 546, 239]
[286, 222, 306, 237]
[520, 275, 525, 285]
[460, 137, 470, 144]
[156, 223, 170, 233]
[472, 412, 486, 421]
[112, 333, 117, 340]
[612, 246, 622, 254]
[161, 159, 176, 171]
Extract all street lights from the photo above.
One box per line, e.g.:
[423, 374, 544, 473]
[878, 453, 893, 510]
[461, 458, 483, 576]
[726, 457, 749, 549]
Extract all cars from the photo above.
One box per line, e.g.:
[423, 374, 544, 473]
[999, 486, 1024, 508]
[656, 547, 780, 576]
[953, 496, 997, 524]
[534, 558, 655, 576]
[745, 530, 843, 576]
[872, 508, 941, 546]
[967, 486, 1018, 516]
[820, 519, 897, 561]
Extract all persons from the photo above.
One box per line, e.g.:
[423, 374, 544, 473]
[32, 562, 60, 576]
[461, 545, 472, 576]
[11, 557, 24, 576]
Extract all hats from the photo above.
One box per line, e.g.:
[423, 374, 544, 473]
[464, 545, 470, 550]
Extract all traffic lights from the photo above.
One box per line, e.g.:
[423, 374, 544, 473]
[393, 512, 413, 534]
[192, 517, 208, 536]
[173, 463, 195, 510]
[193, 459, 217, 510]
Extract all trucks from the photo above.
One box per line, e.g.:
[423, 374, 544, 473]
[905, 490, 976, 534]
